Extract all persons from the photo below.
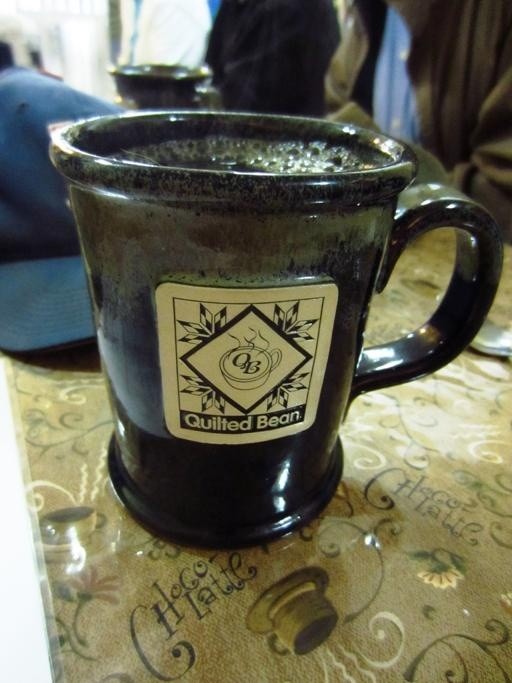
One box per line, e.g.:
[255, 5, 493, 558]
[325, 0, 511, 243]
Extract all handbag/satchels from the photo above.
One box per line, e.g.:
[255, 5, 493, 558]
[1, 65, 129, 356]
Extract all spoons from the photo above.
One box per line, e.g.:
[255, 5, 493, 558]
[470, 319, 512, 359]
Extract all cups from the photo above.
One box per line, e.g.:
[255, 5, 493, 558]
[107, 62, 211, 110]
[50, 109, 504, 551]
